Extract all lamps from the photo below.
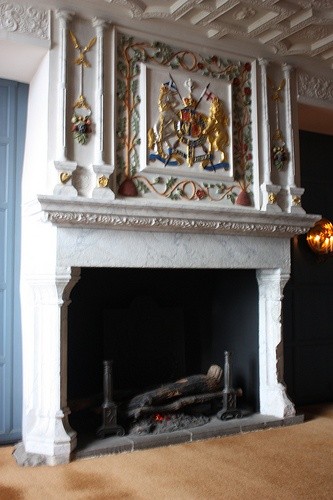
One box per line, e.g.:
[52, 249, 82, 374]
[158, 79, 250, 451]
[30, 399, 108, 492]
[305, 217, 333, 254]
